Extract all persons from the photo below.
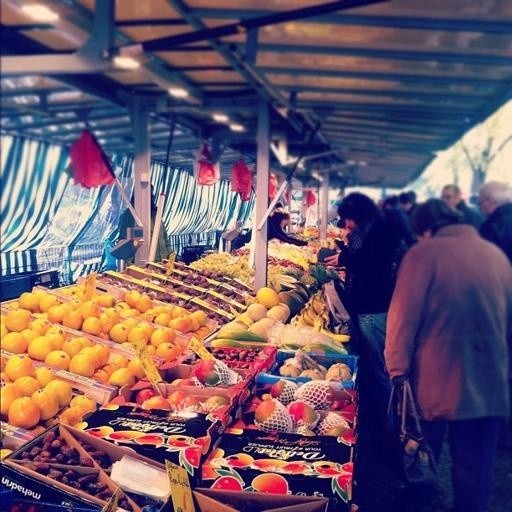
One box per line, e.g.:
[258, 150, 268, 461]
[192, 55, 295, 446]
[231, 203, 309, 254]
[119, 184, 172, 264]
[325, 181, 511, 510]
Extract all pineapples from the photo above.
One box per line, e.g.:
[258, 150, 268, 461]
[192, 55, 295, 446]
[280, 263, 326, 288]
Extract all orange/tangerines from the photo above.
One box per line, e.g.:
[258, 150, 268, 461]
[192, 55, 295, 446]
[0, 277, 354, 436]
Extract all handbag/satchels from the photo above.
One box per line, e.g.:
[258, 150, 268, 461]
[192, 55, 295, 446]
[383, 379, 439, 490]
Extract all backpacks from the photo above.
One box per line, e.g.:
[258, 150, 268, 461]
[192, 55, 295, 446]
[324, 280, 360, 335]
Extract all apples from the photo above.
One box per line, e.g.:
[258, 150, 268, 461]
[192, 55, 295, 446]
[85, 426, 353, 498]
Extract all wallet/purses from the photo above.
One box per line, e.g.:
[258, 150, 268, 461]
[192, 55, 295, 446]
[317, 247, 338, 262]
